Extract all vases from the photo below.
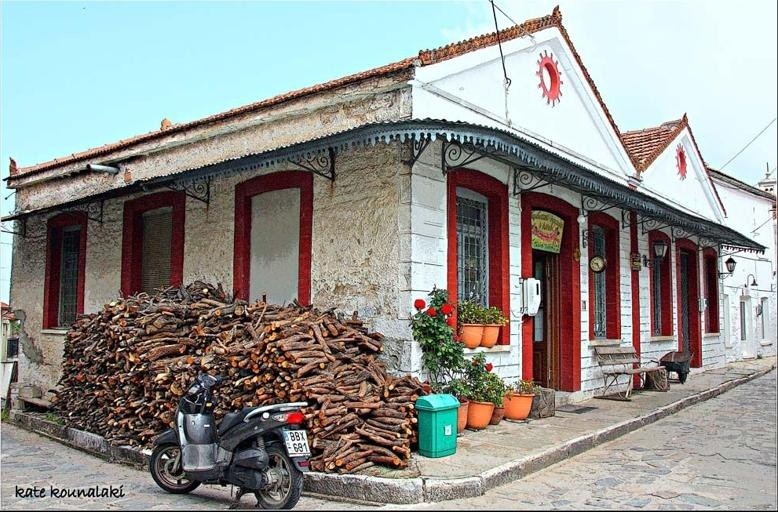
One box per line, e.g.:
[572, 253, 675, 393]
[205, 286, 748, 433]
[469, 399, 494, 431]
[456, 396, 468, 434]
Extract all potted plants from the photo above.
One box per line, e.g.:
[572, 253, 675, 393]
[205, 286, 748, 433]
[454, 297, 483, 349]
[503, 378, 541, 420]
[482, 306, 510, 347]
[489, 378, 505, 426]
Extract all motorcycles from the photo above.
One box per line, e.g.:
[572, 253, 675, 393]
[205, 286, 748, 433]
[150, 373, 313, 510]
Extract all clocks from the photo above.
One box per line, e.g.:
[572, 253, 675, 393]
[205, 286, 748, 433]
[590, 256, 607, 273]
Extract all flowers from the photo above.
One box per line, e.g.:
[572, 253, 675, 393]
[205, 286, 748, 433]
[469, 350, 503, 402]
[408, 283, 468, 400]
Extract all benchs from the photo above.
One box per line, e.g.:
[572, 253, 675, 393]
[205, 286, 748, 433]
[594, 345, 661, 401]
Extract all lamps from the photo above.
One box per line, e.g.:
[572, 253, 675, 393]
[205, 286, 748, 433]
[743, 273, 757, 287]
[717, 256, 736, 277]
[755, 303, 762, 318]
[577, 207, 587, 225]
[642, 240, 668, 267]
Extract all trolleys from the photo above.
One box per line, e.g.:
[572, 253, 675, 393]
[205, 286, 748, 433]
[651, 350, 694, 385]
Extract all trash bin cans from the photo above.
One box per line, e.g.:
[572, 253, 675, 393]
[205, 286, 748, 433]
[415, 393, 461, 458]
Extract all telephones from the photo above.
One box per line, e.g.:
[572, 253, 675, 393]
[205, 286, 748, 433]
[527, 278, 541, 316]
